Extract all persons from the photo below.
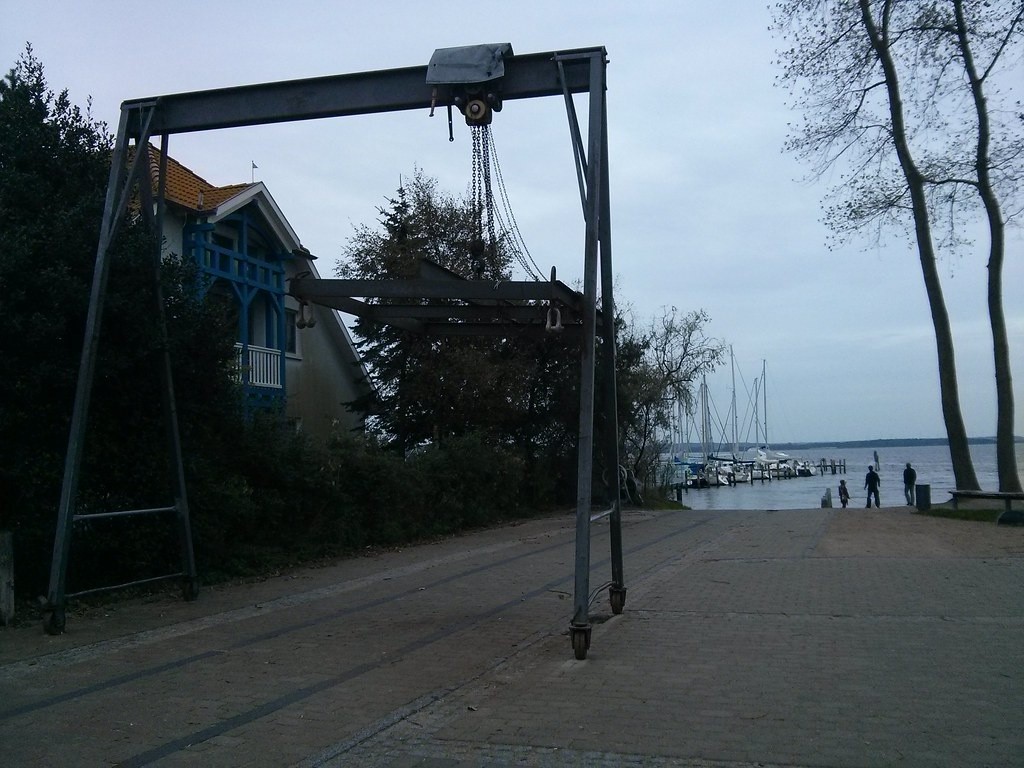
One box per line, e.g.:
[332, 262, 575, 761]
[838, 480, 850, 508]
[863, 465, 881, 509]
[902, 463, 917, 506]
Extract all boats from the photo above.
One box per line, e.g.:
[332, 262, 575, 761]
[654, 343, 818, 487]
